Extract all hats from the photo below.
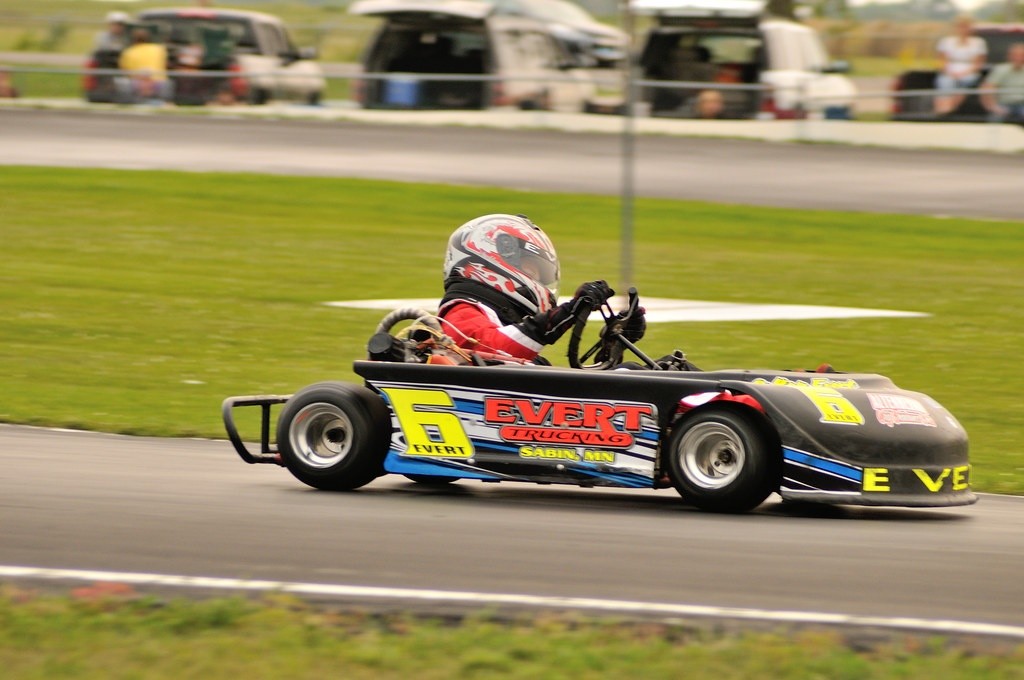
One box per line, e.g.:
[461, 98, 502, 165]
[104, 10, 129, 23]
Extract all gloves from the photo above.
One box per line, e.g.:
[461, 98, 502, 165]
[568, 280, 611, 315]
[615, 306, 645, 347]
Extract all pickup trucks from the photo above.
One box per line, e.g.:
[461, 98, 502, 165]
[888, 16, 1024, 129]
[82, 6, 324, 107]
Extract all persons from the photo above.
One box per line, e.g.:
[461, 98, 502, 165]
[434, 212, 648, 369]
[80, 0, 1023, 124]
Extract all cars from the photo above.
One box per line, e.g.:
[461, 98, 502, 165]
[343, 1, 633, 118]
[634, 7, 857, 120]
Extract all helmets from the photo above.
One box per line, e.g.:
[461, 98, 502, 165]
[442, 214, 560, 317]
